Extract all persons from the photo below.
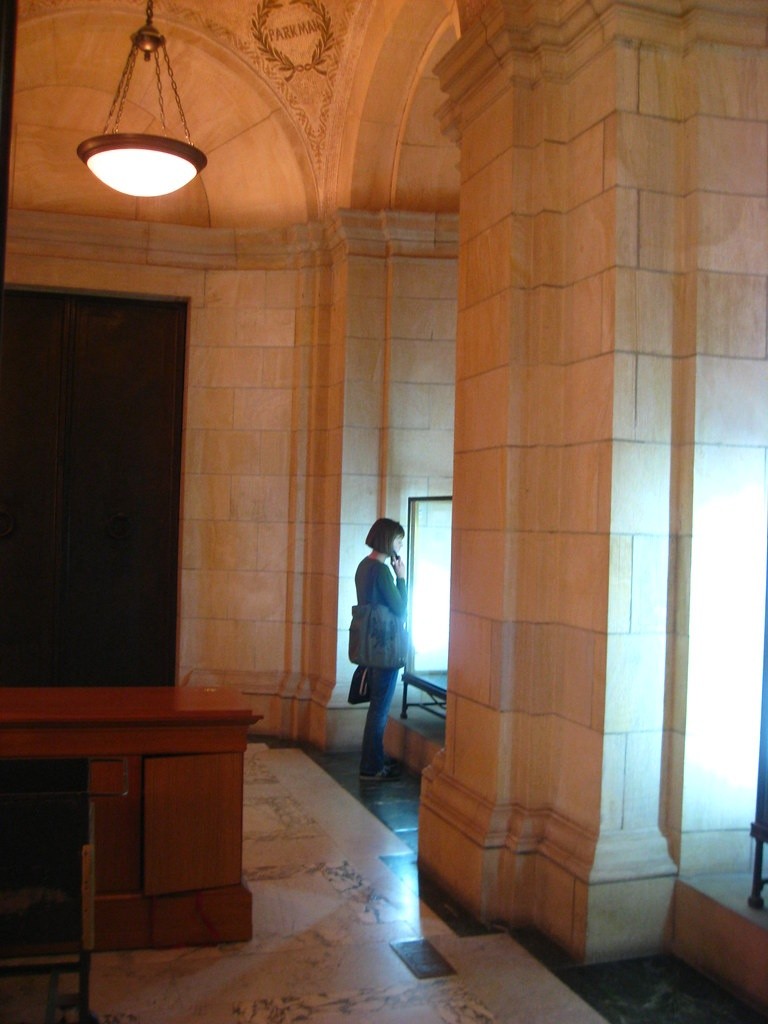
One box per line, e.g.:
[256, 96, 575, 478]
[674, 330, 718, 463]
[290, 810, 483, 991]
[348, 518, 411, 781]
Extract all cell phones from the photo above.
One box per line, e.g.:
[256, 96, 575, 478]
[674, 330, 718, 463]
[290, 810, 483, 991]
[391, 551, 398, 560]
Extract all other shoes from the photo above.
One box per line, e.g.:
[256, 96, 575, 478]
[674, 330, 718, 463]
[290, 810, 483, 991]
[384, 757, 399, 767]
[359, 767, 401, 780]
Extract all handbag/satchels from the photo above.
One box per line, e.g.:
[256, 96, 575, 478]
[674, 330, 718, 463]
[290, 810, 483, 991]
[348, 665, 371, 705]
[348, 563, 408, 670]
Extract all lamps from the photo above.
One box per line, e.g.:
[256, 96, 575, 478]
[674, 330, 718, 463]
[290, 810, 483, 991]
[76, 0, 206, 197]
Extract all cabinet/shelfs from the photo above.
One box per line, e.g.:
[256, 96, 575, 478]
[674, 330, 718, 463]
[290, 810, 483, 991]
[0, 686, 265, 952]
